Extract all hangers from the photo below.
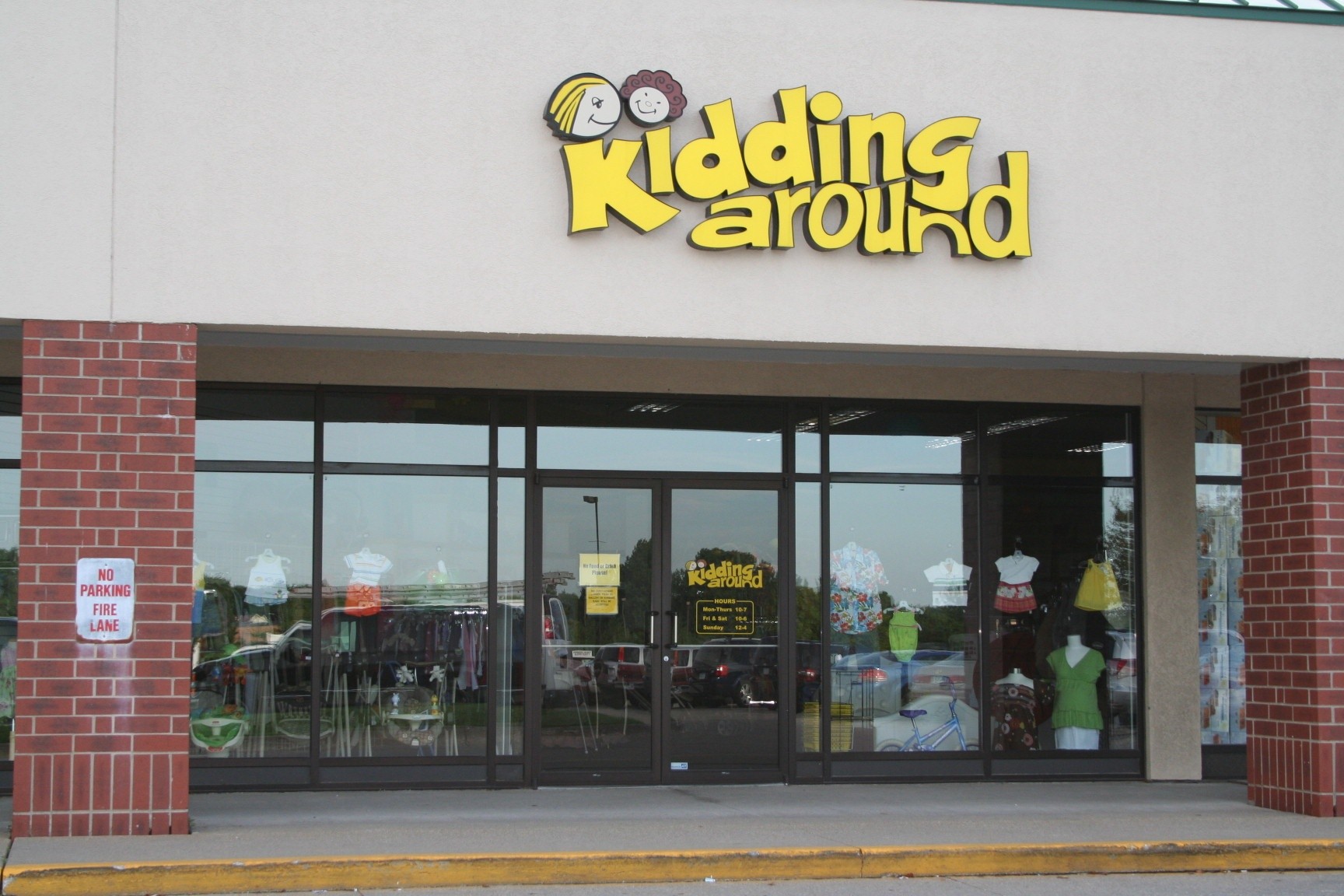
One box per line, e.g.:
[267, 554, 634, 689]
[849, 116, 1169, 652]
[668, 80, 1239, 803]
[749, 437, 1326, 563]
[1012, 539, 1023, 559]
[884, 599, 924, 631]
[1077, 538, 1119, 570]
[245, 536, 291, 563]
[945, 544, 953, 563]
[193, 546, 215, 569]
[342, 534, 372, 560]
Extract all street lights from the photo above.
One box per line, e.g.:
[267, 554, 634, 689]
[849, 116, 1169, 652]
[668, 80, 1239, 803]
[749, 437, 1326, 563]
[584, 496, 600, 554]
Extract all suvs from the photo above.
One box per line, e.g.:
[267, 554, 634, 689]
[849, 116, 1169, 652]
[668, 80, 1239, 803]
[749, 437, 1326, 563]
[498, 599, 590, 707]
[191, 603, 488, 716]
[1198, 628, 1247, 745]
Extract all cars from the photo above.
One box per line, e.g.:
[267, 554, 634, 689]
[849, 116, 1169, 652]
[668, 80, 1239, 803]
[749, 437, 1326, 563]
[826, 650, 979, 715]
[691, 636, 849, 712]
[593, 642, 701, 709]
[1103, 630, 1144, 728]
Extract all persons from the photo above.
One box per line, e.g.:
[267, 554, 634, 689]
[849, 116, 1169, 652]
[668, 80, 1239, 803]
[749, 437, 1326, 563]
[991, 668, 1049, 751]
[1047, 635, 1106, 750]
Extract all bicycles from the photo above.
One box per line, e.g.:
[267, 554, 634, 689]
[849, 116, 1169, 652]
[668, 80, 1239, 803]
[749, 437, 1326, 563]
[871, 675, 979, 751]
